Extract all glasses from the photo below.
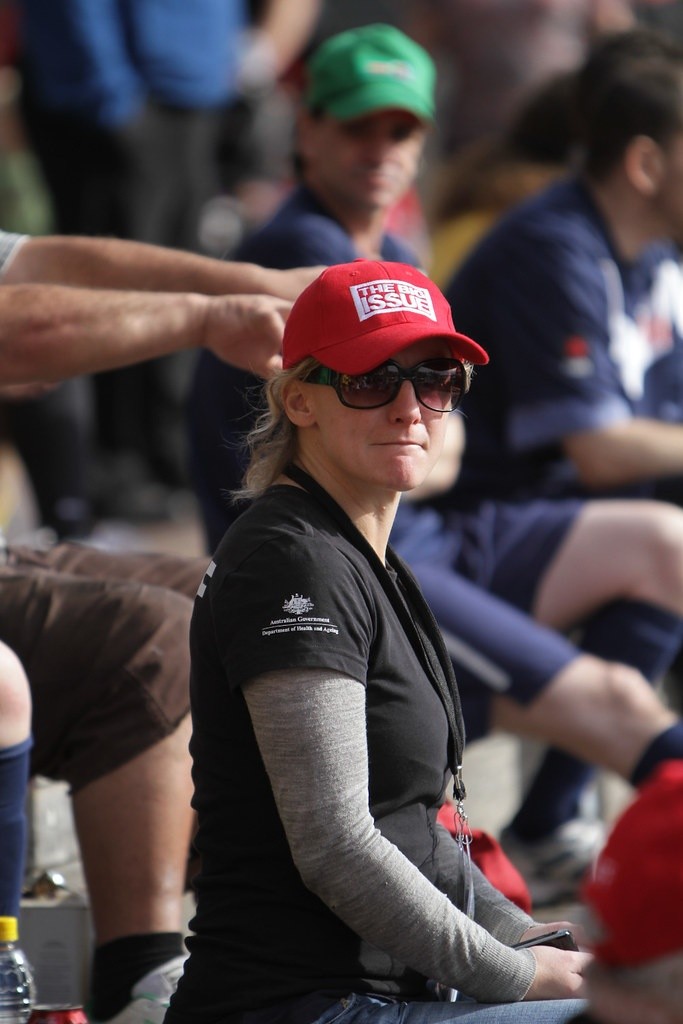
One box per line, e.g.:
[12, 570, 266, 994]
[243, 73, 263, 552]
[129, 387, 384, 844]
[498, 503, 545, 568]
[303, 358, 467, 413]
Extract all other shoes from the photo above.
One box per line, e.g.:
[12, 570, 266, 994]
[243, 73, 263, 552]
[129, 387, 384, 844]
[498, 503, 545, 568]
[498, 819, 616, 902]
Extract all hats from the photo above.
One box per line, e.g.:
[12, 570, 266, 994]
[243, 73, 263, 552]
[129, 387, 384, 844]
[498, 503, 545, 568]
[282, 258, 489, 369]
[302, 22, 436, 130]
[586, 760, 683, 968]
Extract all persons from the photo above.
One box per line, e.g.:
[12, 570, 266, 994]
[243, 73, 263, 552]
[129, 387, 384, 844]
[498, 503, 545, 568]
[164, 258, 595, 1024]
[442, 27, 683, 907]
[0, 0, 683, 543]
[565, 762, 683, 1024]
[1, 231, 324, 1024]
[184, 24, 683, 792]
[1, 641, 32, 917]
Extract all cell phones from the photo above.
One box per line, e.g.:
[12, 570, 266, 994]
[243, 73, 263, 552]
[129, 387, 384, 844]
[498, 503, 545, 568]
[511, 928, 579, 953]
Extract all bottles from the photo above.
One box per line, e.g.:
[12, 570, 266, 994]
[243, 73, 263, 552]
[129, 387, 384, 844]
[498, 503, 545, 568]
[0, 916, 36, 1023]
